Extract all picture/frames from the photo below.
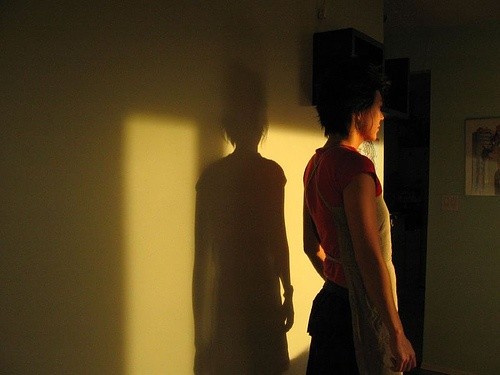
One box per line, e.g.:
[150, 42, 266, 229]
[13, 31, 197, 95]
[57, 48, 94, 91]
[465, 117, 500, 198]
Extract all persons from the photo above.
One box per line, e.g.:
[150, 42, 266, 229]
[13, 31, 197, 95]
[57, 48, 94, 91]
[304, 65, 416, 375]
